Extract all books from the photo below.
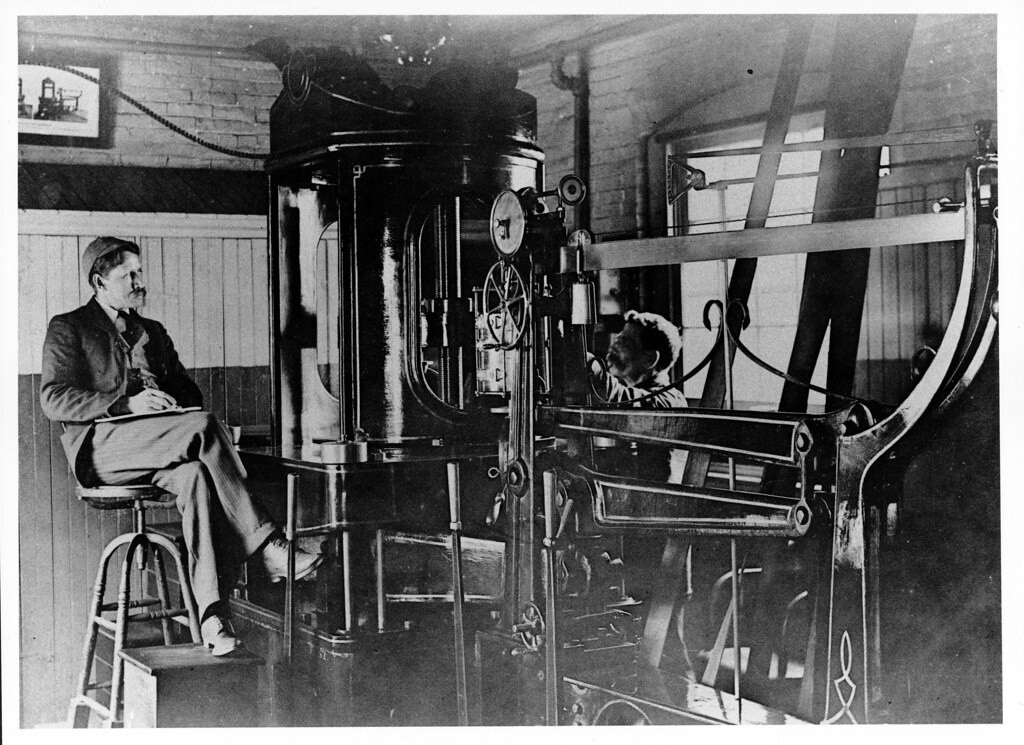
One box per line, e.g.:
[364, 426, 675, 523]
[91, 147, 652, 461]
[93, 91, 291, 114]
[95, 407, 201, 422]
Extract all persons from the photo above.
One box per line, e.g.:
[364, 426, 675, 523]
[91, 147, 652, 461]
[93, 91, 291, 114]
[39, 236, 323, 656]
[587, 311, 694, 681]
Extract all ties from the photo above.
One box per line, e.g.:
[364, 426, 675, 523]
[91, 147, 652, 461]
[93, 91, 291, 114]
[118, 312, 139, 346]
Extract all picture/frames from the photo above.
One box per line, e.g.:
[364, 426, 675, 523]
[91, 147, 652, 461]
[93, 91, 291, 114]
[18, 46, 118, 150]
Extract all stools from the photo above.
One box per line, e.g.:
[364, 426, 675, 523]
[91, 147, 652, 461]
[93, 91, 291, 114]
[66, 422, 203, 730]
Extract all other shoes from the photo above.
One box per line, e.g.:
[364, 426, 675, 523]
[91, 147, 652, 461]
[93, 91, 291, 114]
[200, 614, 248, 658]
[262, 536, 327, 589]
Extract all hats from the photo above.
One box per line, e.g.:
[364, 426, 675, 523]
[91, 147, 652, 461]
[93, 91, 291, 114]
[82, 236, 128, 286]
